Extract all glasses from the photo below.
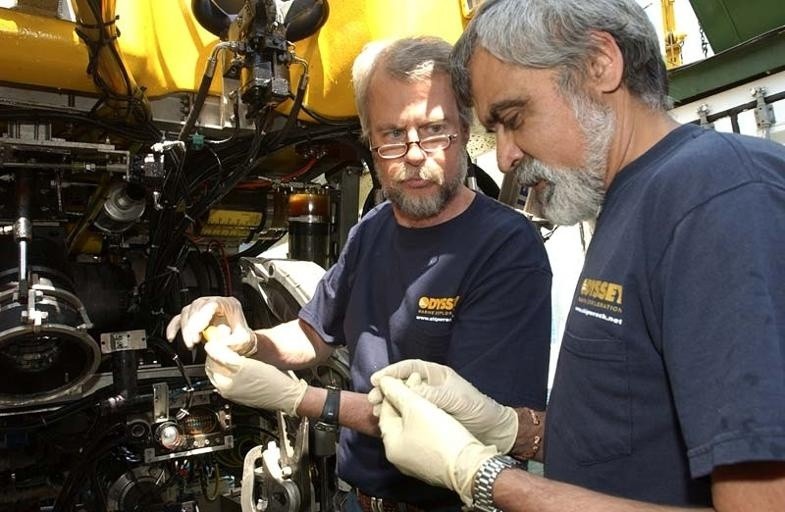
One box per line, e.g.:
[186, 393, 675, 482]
[367, 116, 463, 160]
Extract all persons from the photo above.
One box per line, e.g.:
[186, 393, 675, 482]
[365, 0, 785, 511]
[166, 32, 552, 509]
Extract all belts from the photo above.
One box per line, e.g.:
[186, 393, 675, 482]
[353, 485, 427, 512]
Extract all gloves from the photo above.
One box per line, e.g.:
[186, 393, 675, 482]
[202, 341, 304, 421]
[378, 376, 500, 508]
[163, 293, 257, 356]
[367, 357, 518, 454]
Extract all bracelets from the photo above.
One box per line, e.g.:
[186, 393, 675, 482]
[315, 382, 345, 435]
[472, 456, 522, 511]
[512, 406, 543, 463]
